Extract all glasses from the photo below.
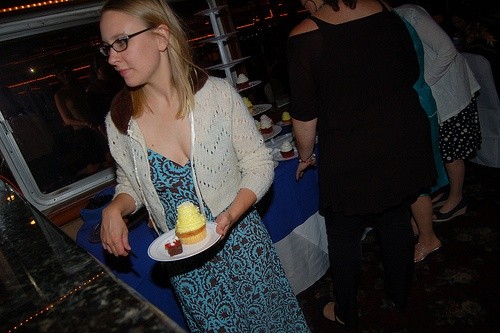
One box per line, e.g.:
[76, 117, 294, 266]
[99, 28, 152, 56]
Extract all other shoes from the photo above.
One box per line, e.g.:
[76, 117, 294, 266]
[317, 296, 339, 324]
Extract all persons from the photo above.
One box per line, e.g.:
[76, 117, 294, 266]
[287, 0, 433, 328]
[382, 10, 450, 262]
[54, 54, 119, 174]
[100, 0, 311, 333]
[381, 0, 482, 222]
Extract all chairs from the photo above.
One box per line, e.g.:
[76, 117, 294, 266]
[463, 53, 500, 171]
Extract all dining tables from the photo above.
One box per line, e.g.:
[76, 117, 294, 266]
[76, 125, 331, 333]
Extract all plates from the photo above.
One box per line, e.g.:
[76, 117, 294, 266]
[278, 121, 291, 126]
[85, 194, 113, 209]
[272, 151, 298, 161]
[88, 210, 147, 245]
[147, 222, 222, 262]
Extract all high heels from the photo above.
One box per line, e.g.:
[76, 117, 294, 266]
[432, 190, 449, 209]
[412, 236, 443, 263]
[432, 197, 469, 222]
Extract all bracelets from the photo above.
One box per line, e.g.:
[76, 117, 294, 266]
[299, 153, 316, 163]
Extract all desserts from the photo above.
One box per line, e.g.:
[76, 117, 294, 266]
[282, 112, 292, 125]
[165, 201, 207, 256]
[280, 141, 295, 158]
[260, 114, 274, 134]
[254, 120, 262, 135]
[236, 74, 249, 90]
[242, 97, 255, 114]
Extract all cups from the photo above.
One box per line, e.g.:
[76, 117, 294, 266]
[314, 132, 318, 144]
